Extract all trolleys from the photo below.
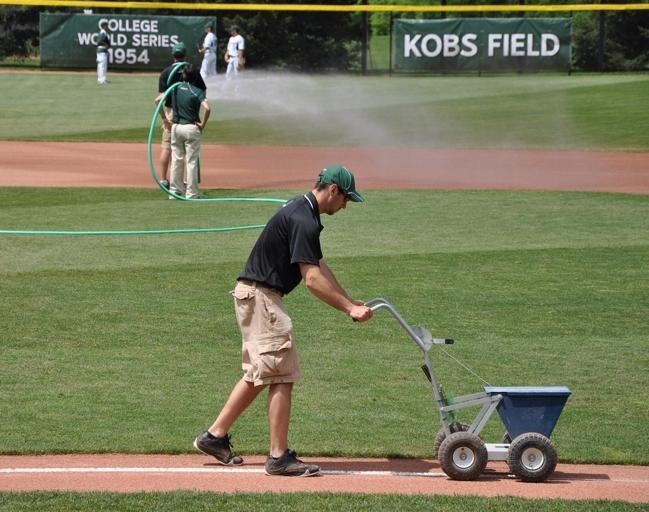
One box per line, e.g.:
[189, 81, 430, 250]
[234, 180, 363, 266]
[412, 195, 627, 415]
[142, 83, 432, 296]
[347, 292, 577, 486]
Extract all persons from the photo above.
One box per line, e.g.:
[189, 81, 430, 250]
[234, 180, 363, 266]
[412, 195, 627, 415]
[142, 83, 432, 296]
[95, 21, 112, 84]
[153, 63, 211, 200]
[160, 42, 193, 189]
[198, 24, 219, 81]
[192, 164, 373, 476]
[224, 24, 247, 96]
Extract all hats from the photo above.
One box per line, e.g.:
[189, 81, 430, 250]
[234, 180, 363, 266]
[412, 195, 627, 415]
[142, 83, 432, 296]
[229, 24, 239, 30]
[172, 42, 186, 57]
[204, 22, 213, 28]
[318, 164, 365, 202]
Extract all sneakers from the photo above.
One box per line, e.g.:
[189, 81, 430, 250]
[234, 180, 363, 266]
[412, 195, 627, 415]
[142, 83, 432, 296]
[161, 181, 208, 200]
[264, 448, 321, 478]
[193, 431, 244, 466]
